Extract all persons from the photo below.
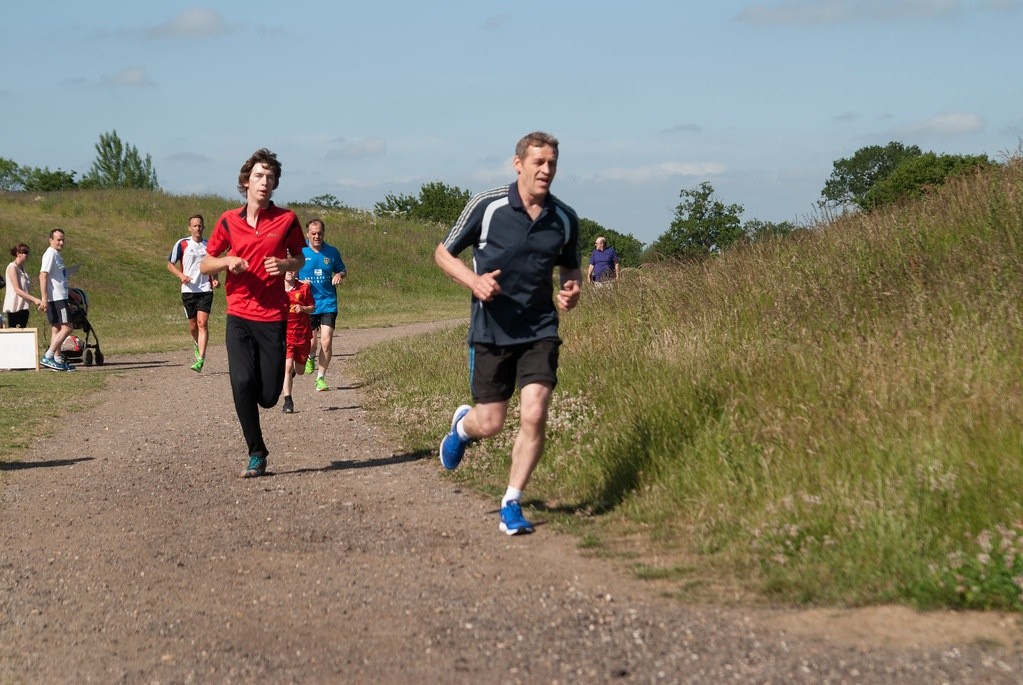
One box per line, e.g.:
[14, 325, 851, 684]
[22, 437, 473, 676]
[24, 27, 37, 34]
[3, 243, 41, 328]
[200, 150, 307, 479]
[587, 236, 620, 288]
[435, 131, 582, 536]
[298, 219, 347, 391]
[283, 270, 316, 412]
[39, 229, 76, 372]
[168, 215, 221, 372]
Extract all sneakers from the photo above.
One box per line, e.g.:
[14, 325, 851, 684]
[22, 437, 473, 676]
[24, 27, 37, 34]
[497, 499, 533, 536]
[314, 376, 329, 391]
[240, 455, 267, 477]
[291, 369, 296, 378]
[439, 405, 472, 470]
[283, 400, 293, 413]
[194, 344, 200, 359]
[305, 353, 316, 374]
[191, 358, 204, 373]
[53, 361, 76, 371]
[40, 355, 65, 370]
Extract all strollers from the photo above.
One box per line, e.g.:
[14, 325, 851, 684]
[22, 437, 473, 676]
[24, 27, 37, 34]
[46, 288, 104, 367]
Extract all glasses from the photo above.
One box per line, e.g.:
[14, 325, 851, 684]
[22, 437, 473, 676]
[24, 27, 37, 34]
[19, 250, 29, 254]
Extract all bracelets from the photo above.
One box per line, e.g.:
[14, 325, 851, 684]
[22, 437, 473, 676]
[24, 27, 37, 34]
[340, 273, 343, 277]
